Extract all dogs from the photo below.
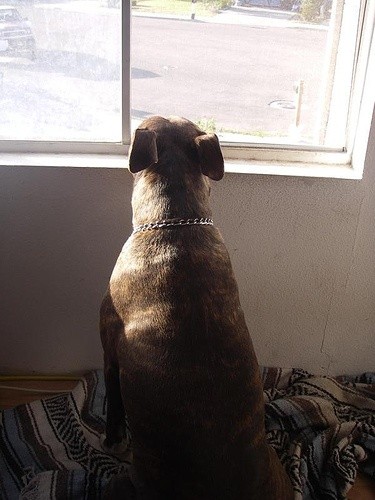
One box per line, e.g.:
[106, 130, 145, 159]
[99, 115, 295, 500]
